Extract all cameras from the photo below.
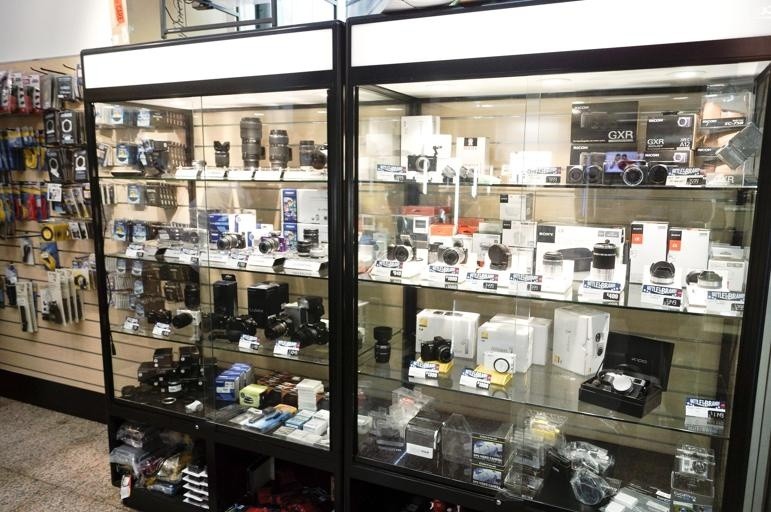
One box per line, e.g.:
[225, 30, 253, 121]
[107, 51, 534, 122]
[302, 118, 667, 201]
[290, 321, 329, 349]
[672, 500, 696, 512]
[227, 314, 258, 343]
[420, 336, 455, 363]
[483, 351, 516, 374]
[672, 152, 688, 164]
[566, 160, 678, 186]
[580, 112, 609, 130]
[404, 416, 444, 453]
[597, 368, 648, 399]
[199, 311, 225, 333]
[408, 145, 443, 173]
[258, 231, 289, 255]
[264, 316, 294, 340]
[437, 239, 468, 266]
[387, 234, 417, 262]
[579, 152, 607, 168]
[216, 231, 247, 251]
[672, 444, 716, 482]
[677, 116, 692, 128]
